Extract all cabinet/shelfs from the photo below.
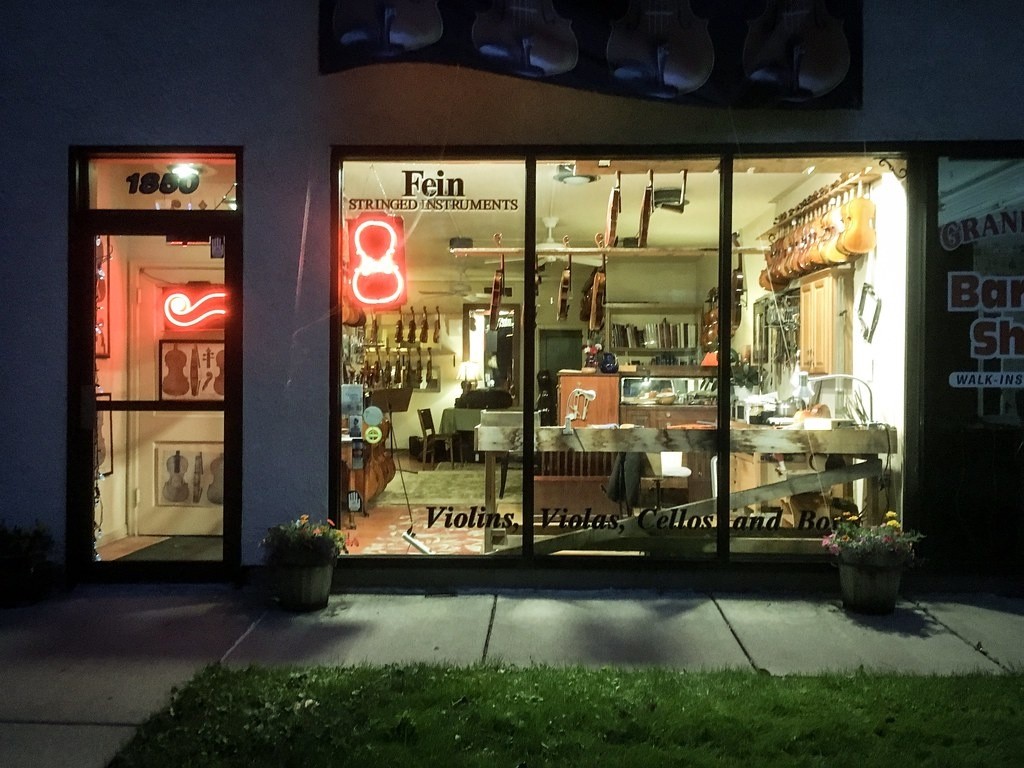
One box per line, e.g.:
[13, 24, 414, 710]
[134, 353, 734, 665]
[605, 302, 703, 365]
[556, 372, 621, 428]
[797, 266, 856, 377]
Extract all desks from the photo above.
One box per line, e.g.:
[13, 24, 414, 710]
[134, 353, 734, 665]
[441, 407, 517, 435]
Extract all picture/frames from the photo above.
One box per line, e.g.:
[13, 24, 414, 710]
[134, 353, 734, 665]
[95, 392, 114, 476]
[95, 235, 111, 359]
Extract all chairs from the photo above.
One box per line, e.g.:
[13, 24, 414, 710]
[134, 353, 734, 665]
[417, 408, 465, 470]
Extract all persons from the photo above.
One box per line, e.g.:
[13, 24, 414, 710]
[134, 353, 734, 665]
[350, 417, 360, 437]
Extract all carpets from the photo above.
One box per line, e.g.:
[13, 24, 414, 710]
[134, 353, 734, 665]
[371, 471, 523, 504]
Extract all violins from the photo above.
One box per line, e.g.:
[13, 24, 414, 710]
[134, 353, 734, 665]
[601, 170, 622, 247]
[589, 232, 609, 330]
[342, 259, 368, 328]
[699, 232, 743, 352]
[355, 414, 396, 507]
[363, 305, 442, 386]
[487, 232, 507, 332]
[555, 235, 574, 322]
[636, 169, 655, 249]
[757, 168, 877, 294]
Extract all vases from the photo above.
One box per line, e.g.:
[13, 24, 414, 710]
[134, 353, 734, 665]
[272, 557, 334, 611]
[836, 561, 906, 613]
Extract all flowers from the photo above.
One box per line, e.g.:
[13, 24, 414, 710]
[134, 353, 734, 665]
[260, 513, 350, 562]
[821, 508, 928, 567]
